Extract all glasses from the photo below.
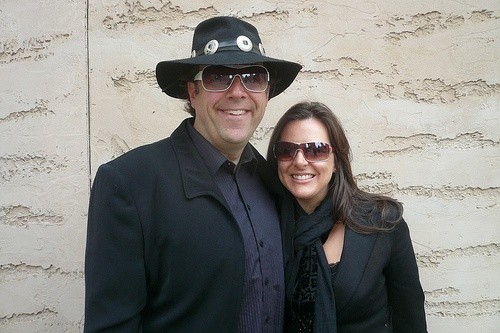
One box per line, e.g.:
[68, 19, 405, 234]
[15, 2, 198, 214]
[193, 64, 270, 92]
[273, 140, 336, 162]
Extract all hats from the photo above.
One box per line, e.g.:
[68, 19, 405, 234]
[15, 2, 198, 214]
[155, 16, 303, 101]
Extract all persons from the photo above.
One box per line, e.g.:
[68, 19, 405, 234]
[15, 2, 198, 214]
[267, 102, 427, 333]
[84, 16, 303, 333]
